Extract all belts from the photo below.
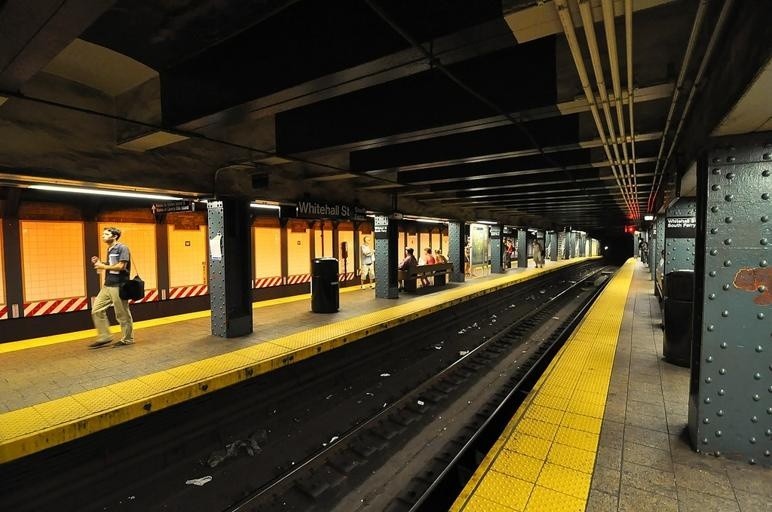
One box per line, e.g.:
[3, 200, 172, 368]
[367, 263, 372, 266]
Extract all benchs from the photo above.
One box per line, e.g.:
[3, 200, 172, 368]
[397, 262, 454, 292]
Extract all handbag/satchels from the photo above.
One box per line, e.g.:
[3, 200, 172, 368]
[118, 275, 145, 301]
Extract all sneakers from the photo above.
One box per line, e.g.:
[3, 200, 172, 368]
[87, 340, 113, 349]
[112, 341, 127, 348]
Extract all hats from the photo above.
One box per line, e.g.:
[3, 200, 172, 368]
[405, 247, 413, 253]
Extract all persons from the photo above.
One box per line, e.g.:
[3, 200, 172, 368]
[638, 237, 648, 262]
[500, 238, 546, 271]
[656, 250, 665, 274]
[86, 226, 134, 350]
[398, 242, 473, 293]
[360, 235, 376, 291]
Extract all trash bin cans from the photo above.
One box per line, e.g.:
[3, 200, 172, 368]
[661, 270, 694, 368]
[312, 258, 340, 312]
